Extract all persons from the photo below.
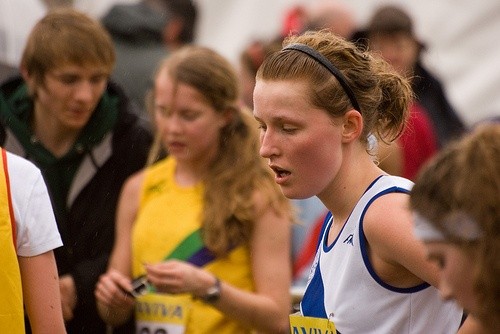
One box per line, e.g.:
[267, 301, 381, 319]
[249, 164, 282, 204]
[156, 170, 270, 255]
[253, 31, 487, 334]
[95, 45, 290, 334]
[0, 10, 167, 334]
[407, 116, 500, 334]
[36, 0, 472, 179]
[0, 147, 67, 334]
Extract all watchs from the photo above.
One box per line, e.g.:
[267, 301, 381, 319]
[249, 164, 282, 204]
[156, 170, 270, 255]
[196, 276, 224, 304]
